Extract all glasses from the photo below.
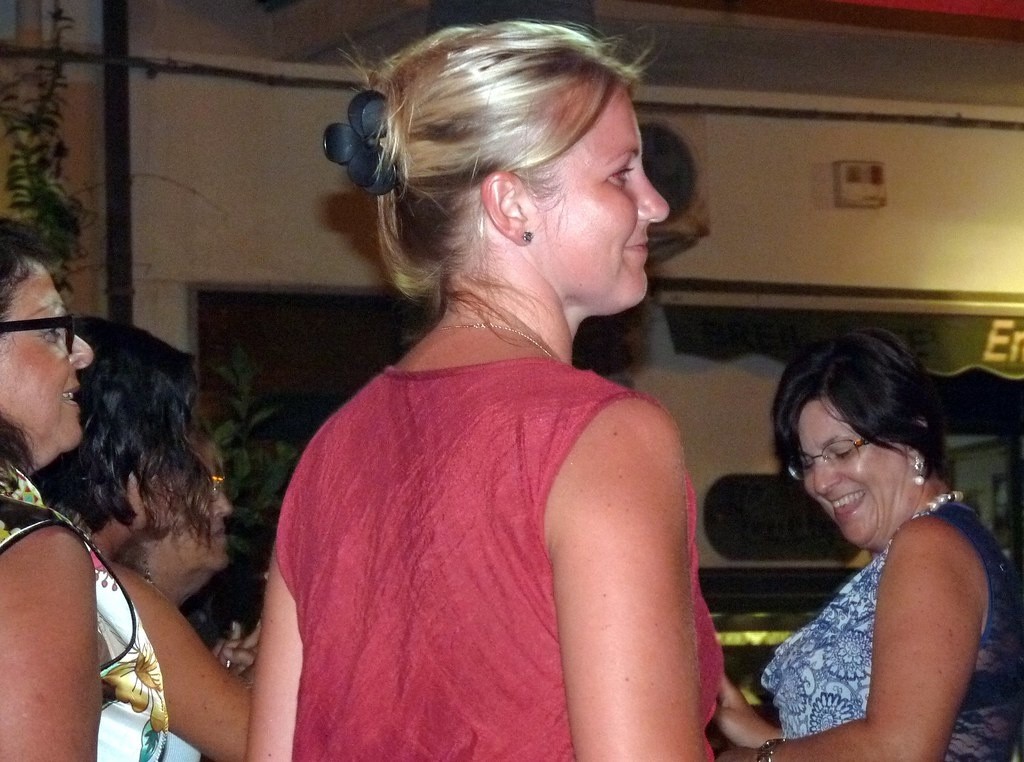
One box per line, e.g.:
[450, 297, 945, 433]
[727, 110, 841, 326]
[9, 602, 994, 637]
[786, 426, 894, 482]
[0, 314, 75, 353]
[205, 476, 226, 503]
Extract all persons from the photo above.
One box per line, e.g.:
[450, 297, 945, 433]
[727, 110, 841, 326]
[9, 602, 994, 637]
[33, 316, 271, 762]
[0, 215, 169, 762]
[246, 21, 722, 761]
[711, 328, 1024, 762]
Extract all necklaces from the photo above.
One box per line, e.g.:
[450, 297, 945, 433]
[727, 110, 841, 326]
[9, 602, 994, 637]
[427, 323, 551, 358]
[927, 492, 963, 513]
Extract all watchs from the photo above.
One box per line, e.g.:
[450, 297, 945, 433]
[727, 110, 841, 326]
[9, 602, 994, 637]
[757, 739, 786, 762]
